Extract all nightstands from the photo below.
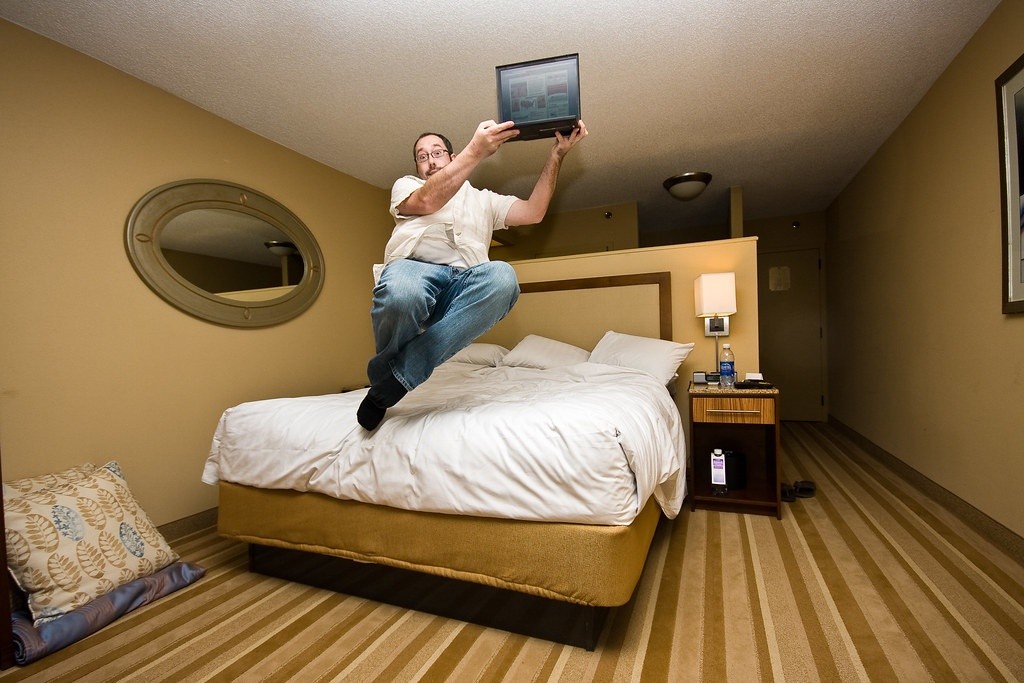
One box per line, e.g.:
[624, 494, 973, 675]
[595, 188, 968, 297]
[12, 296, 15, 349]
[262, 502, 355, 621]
[688, 381, 781, 520]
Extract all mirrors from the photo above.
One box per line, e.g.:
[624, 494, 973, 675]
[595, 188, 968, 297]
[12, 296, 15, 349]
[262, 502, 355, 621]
[123, 178, 326, 328]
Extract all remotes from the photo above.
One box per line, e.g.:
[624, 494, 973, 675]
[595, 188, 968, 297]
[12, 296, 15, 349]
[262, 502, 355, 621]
[734, 381, 773, 389]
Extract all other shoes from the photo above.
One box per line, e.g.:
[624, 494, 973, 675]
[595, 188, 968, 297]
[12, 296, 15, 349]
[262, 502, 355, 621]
[781, 482, 796, 502]
[794, 481, 816, 497]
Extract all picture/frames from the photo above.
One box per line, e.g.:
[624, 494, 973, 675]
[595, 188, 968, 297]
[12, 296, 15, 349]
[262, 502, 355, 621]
[994, 53, 1024, 313]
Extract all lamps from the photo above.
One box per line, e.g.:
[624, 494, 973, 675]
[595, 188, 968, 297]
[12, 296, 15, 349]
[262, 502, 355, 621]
[695, 271, 737, 374]
[265, 241, 295, 256]
[663, 171, 713, 200]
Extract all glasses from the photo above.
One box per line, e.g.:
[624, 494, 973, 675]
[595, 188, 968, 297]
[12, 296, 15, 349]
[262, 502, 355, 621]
[415, 149, 450, 163]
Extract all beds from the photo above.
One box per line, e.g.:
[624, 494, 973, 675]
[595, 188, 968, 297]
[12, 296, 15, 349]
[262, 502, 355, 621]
[202, 272, 686, 652]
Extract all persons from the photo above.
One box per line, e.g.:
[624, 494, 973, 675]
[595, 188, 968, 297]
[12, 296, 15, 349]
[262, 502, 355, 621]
[357, 118, 589, 431]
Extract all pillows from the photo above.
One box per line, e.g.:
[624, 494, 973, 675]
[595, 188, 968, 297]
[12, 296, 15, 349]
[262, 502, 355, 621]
[2, 464, 100, 503]
[446, 343, 511, 366]
[3, 460, 182, 630]
[587, 330, 695, 387]
[501, 334, 591, 371]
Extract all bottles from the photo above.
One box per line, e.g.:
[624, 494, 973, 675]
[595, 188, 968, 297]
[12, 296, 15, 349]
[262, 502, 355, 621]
[720, 344, 734, 388]
[711, 449, 727, 496]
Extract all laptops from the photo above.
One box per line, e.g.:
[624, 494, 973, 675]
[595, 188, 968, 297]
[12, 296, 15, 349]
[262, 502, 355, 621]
[495, 53, 580, 143]
